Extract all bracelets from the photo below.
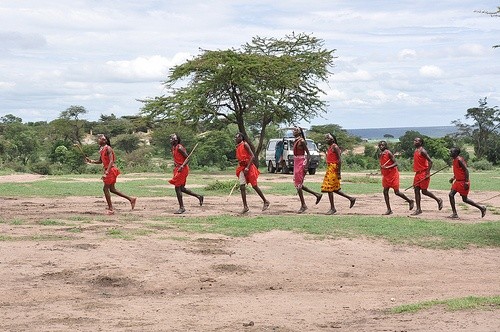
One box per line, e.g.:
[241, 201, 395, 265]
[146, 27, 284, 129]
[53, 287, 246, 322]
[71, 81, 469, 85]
[180, 166, 183, 168]
[426, 172, 430, 174]
[465, 182, 470, 185]
[105, 171, 108, 175]
[245, 168, 249, 170]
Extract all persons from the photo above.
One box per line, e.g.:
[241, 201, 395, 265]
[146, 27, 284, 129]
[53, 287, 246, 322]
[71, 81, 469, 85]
[86, 135, 136, 210]
[411, 137, 443, 215]
[378, 141, 414, 215]
[235, 132, 270, 214]
[445, 147, 486, 219]
[321, 132, 356, 215]
[293, 127, 323, 213]
[168, 134, 203, 214]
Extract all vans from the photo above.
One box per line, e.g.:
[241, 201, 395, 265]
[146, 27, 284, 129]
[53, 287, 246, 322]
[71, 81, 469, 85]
[265, 126, 321, 175]
